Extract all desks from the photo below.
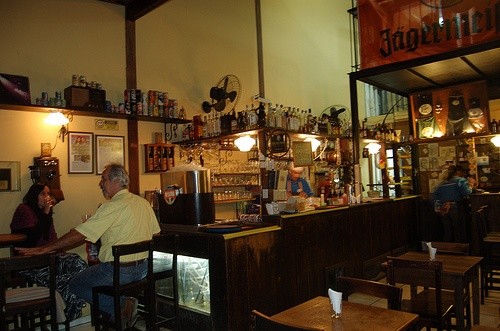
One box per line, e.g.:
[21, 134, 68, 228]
[382, 251, 484, 331]
[272, 296, 420, 331]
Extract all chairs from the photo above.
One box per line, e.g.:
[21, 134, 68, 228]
[92, 232, 181, 331]
[423, 241, 470, 330]
[248, 310, 324, 331]
[385, 256, 441, 331]
[476, 204, 500, 302]
[10, 231, 69, 331]
[334, 276, 402, 311]
[0, 251, 58, 331]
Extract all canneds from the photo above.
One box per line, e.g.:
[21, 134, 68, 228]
[72, 74, 102, 89]
[36, 90, 66, 108]
[106, 88, 179, 119]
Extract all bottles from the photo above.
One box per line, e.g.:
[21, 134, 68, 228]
[213, 190, 239, 200]
[191, 102, 340, 147]
[491, 119, 497, 133]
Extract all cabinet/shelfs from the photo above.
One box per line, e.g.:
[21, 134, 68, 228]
[145, 125, 500, 206]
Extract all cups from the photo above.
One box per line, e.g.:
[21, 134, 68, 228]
[329, 304, 343, 318]
[45, 194, 55, 207]
[429, 253, 437, 261]
[210, 160, 260, 185]
[82, 215, 91, 223]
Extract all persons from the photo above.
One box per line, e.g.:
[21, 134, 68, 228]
[10, 180, 88, 321]
[432, 165, 476, 242]
[286, 161, 312, 198]
[14, 164, 162, 327]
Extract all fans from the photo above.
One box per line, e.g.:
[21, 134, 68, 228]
[319, 104, 351, 130]
[202, 74, 241, 115]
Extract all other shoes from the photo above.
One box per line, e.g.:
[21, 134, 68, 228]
[113, 297, 138, 331]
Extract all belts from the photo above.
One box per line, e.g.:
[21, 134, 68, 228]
[108, 258, 147, 267]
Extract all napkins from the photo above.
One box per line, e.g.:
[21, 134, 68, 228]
[328, 288, 343, 312]
[426, 242, 435, 257]
